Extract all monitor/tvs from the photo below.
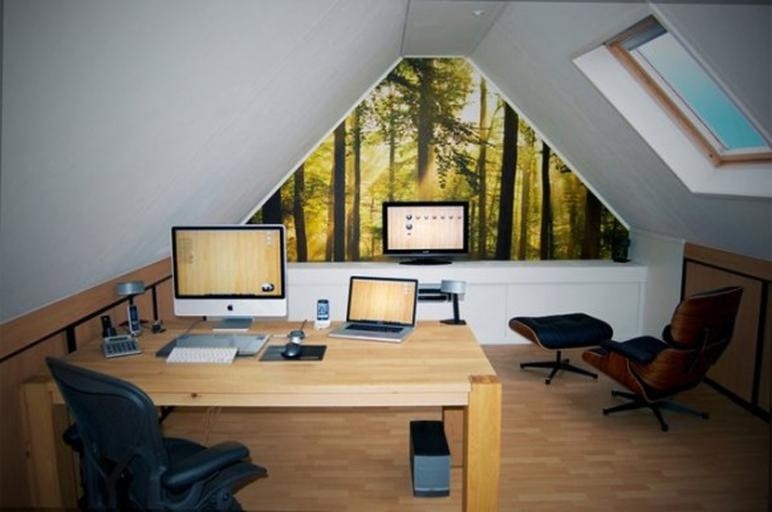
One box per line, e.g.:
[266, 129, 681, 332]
[168, 224, 289, 334]
[382, 201, 469, 265]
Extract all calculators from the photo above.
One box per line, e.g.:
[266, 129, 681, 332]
[102, 334, 142, 358]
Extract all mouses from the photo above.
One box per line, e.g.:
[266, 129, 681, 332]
[327, 275, 419, 344]
[283, 342, 301, 357]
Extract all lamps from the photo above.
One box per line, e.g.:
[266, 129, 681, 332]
[439, 278, 467, 326]
[114, 279, 147, 337]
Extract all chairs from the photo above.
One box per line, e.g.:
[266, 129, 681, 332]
[44, 353, 269, 511]
[508, 283, 744, 435]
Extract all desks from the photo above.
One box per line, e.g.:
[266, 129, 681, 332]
[18, 319, 506, 512]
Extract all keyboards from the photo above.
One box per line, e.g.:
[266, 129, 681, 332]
[165, 346, 238, 365]
